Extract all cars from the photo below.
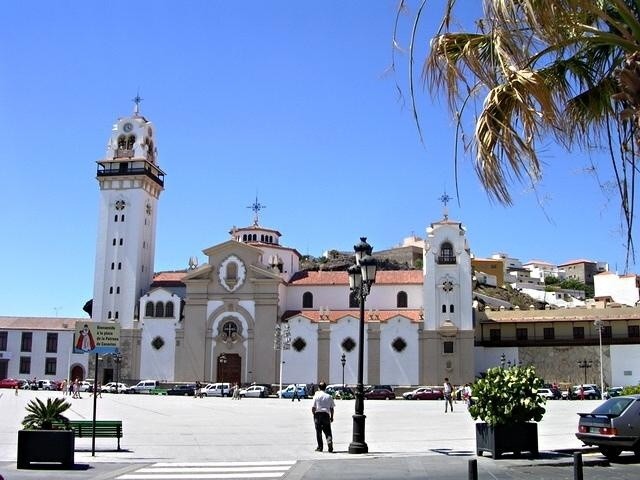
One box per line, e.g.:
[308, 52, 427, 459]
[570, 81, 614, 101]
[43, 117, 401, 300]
[238, 386, 269, 398]
[552, 383, 624, 400]
[402, 387, 432, 400]
[364, 388, 396, 400]
[574, 393, 640, 459]
[535, 388, 555, 400]
[0, 377, 130, 393]
[413, 389, 443, 401]
[364, 384, 395, 392]
[165, 383, 196, 396]
[275, 383, 311, 399]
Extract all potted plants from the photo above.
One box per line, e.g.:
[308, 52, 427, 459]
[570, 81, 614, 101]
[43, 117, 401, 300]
[462, 352, 551, 460]
[14, 394, 80, 472]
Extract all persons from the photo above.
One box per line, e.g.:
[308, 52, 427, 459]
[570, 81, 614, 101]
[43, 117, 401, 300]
[27, 377, 102, 399]
[312, 381, 335, 452]
[193, 380, 203, 398]
[292, 384, 300, 402]
[580, 384, 584, 400]
[230, 381, 241, 399]
[567, 387, 572, 400]
[444, 378, 453, 412]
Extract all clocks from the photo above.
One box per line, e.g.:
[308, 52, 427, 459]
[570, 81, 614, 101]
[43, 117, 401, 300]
[122, 123, 133, 131]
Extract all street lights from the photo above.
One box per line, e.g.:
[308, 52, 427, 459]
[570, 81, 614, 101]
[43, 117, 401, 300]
[593, 319, 606, 399]
[219, 351, 228, 397]
[344, 234, 381, 455]
[577, 358, 593, 384]
[340, 353, 348, 386]
[272, 323, 293, 398]
[500, 353, 523, 370]
[112, 351, 123, 393]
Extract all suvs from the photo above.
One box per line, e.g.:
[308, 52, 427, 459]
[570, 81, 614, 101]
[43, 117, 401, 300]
[194, 382, 232, 398]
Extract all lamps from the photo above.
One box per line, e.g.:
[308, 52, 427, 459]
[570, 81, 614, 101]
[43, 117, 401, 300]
[324, 305, 331, 320]
[374, 304, 381, 320]
[318, 304, 325, 321]
[419, 304, 424, 320]
[368, 306, 374, 321]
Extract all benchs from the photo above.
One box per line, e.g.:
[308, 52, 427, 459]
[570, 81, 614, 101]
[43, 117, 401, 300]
[48, 420, 127, 454]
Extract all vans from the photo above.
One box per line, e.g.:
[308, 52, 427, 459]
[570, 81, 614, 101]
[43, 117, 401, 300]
[127, 380, 160, 395]
